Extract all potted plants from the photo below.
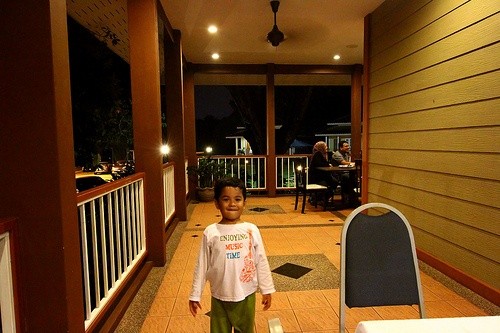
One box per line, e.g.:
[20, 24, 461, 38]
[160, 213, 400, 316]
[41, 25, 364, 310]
[185, 153, 236, 203]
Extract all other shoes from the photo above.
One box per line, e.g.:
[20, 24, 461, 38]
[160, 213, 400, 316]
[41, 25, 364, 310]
[308, 196, 316, 206]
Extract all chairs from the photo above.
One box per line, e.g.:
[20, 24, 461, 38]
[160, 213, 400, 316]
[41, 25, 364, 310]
[350, 160, 362, 207]
[294, 158, 329, 214]
[338, 202, 427, 333]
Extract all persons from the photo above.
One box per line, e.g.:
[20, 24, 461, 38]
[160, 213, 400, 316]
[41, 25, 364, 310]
[188, 177, 275, 333]
[307, 141, 355, 207]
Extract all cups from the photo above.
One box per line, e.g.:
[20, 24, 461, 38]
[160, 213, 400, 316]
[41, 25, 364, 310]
[351, 163, 355, 166]
[339, 165, 347, 167]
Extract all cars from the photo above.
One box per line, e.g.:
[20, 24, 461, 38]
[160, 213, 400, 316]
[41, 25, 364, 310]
[75, 159, 135, 224]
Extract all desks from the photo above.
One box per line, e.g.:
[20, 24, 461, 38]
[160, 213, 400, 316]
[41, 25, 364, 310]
[355, 315, 500, 333]
[316, 164, 362, 211]
